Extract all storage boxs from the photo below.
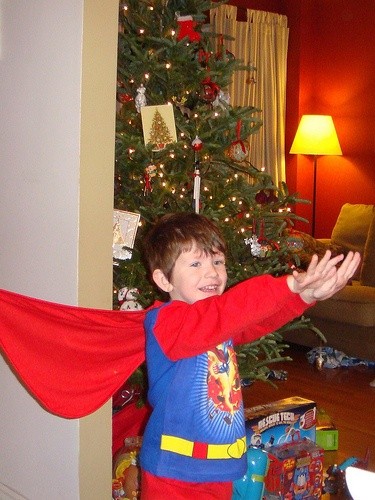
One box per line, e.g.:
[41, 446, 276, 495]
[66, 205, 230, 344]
[316, 404, 339, 450]
[265, 438, 325, 500]
[244, 395, 316, 444]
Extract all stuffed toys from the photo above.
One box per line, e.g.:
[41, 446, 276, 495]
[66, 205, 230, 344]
[117, 288, 143, 310]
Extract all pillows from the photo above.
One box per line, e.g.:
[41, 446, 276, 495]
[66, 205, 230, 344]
[285, 228, 353, 286]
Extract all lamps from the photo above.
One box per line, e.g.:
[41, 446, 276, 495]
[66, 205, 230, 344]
[289, 115, 343, 236]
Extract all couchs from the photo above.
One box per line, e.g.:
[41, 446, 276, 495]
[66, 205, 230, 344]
[282, 203, 375, 363]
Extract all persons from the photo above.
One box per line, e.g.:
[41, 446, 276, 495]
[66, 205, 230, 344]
[139, 213, 362, 500]
